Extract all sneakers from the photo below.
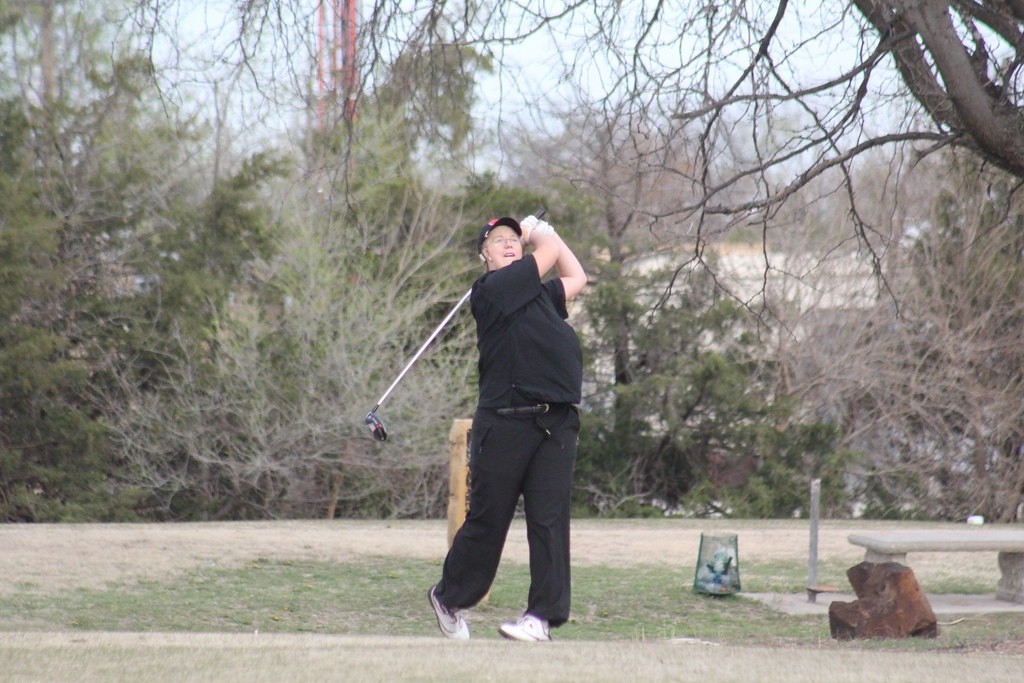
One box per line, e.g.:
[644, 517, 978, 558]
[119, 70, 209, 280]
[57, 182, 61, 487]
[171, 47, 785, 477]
[428, 585, 471, 640]
[498, 614, 553, 641]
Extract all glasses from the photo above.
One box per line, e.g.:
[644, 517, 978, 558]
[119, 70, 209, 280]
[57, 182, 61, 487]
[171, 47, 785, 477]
[484, 235, 522, 247]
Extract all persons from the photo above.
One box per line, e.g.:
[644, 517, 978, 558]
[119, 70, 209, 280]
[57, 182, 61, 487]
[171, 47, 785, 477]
[425, 215, 589, 642]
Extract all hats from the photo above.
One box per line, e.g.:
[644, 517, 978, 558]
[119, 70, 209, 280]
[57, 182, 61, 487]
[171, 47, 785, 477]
[479, 216, 523, 252]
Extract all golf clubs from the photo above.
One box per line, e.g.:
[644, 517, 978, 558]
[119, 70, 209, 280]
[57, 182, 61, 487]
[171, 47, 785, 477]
[364, 285, 472, 446]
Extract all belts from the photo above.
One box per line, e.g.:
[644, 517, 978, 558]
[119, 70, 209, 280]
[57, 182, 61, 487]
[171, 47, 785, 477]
[496, 402, 552, 418]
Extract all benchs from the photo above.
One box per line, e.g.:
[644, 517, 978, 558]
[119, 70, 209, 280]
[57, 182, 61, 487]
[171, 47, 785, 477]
[848, 530, 1024, 605]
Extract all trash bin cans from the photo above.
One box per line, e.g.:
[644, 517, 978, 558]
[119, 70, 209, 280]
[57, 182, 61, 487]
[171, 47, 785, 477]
[693, 531, 743, 598]
